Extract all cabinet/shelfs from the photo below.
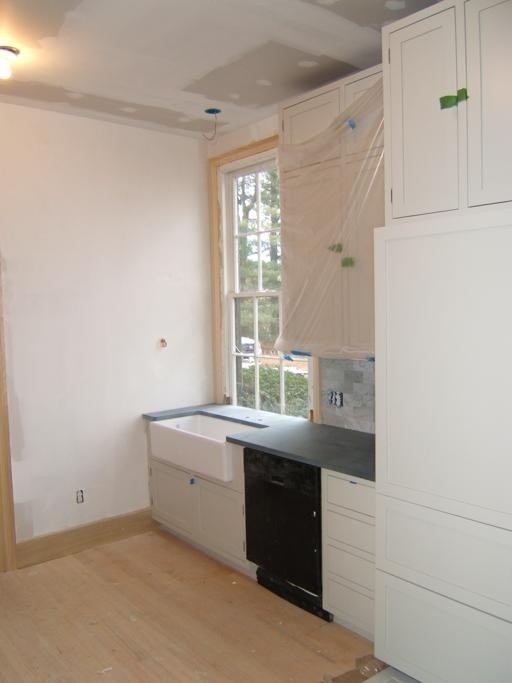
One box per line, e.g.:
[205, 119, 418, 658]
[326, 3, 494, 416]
[321, 470, 376, 639]
[278, 64, 383, 363]
[147, 456, 248, 571]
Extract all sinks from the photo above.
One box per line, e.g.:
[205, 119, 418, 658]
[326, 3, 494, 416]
[149, 414, 258, 482]
